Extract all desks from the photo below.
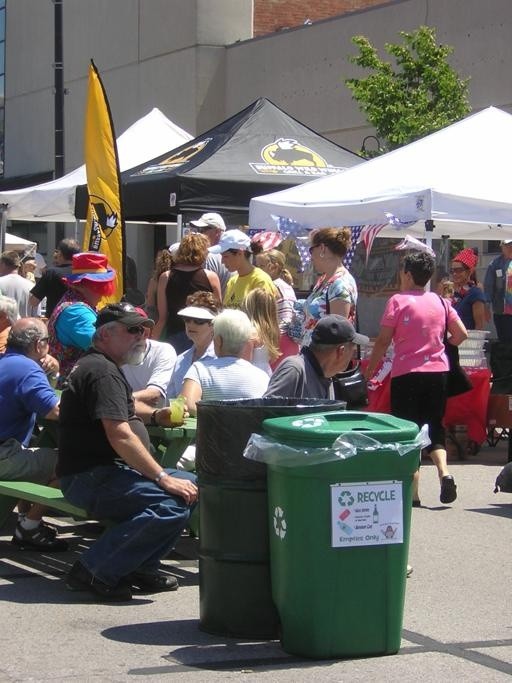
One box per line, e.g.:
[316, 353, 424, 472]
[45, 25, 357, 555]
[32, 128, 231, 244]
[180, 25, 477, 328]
[486, 393, 512, 448]
[351, 359, 490, 460]
[54, 389, 197, 538]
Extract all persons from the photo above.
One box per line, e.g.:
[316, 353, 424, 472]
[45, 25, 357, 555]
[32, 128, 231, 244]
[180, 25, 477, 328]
[365, 251, 468, 508]
[449, 248, 486, 330]
[0, 210, 369, 603]
[484, 240, 512, 394]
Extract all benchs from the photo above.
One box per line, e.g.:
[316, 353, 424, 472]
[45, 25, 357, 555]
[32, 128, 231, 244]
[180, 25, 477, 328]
[0, 480, 133, 602]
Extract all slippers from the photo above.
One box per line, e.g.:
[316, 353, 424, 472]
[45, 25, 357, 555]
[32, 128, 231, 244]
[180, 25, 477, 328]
[440, 475, 457, 503]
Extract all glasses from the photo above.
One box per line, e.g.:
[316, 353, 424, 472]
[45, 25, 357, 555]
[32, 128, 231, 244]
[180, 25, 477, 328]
[184, 316, 209, 324]
[35, 336, 51, 343]
[450, 267, 468, 274]
[127, 324, 144, 334]
[309, 243, 328, 254]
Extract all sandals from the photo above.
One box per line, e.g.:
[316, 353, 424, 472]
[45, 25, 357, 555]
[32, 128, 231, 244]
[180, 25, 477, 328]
[11, 521, 69, 551]
[15, 519, 59, 536]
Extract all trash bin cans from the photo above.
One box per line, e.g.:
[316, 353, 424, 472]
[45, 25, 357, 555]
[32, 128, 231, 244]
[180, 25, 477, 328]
[194, 396, 348, 641]
[261, 409, 421, 661]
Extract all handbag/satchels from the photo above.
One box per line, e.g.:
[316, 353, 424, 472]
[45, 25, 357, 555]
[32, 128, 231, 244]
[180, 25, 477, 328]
[332, 366, 369, 409]
[439, 295, 474, 397]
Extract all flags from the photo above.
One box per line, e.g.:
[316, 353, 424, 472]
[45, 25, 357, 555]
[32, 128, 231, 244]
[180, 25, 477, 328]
[84, 59, 127, 311]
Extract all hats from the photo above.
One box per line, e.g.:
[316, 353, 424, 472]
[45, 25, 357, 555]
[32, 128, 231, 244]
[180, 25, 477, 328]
[206, 228, 254, 254]
[60, 251, 116, 284]
[92, 301, 155, 329]
[312, 313, 370, 346]
[453, 247, 479, 272]
[190, 212, 226, 231]
[176, 303, 217, 320]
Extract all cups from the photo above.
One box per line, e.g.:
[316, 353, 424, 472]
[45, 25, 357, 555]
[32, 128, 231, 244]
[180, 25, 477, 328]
[168, 396, 186, 425]
[441, 280, 456, 297]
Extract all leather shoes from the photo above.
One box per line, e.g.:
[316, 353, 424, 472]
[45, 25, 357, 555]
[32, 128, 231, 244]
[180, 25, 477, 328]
[131, 569, 179, 591]
[71, 559, 132, 601]
